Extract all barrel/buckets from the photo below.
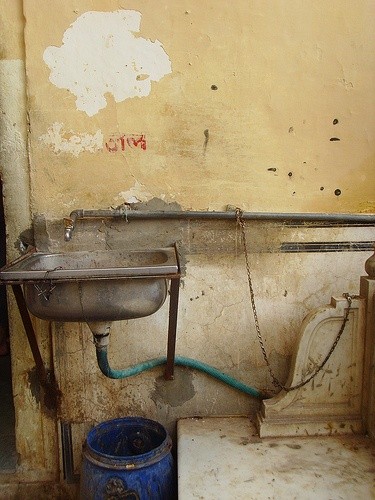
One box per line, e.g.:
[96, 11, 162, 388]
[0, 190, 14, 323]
[80, 417, 178, 500]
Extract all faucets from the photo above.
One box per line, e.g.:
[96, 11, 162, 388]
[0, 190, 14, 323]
[62, 217, 74, 242]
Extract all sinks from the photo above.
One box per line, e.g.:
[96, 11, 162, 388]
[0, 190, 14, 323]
[0, 241, 185, 323]
